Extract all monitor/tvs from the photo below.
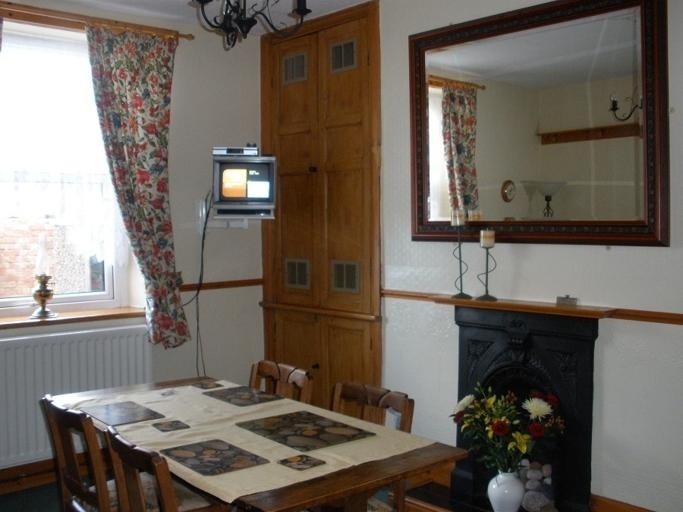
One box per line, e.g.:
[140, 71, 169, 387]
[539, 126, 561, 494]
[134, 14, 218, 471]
[212, 154, 277, 207]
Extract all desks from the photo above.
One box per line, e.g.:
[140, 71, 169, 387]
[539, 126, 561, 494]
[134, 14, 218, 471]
[41, 375, 468, 512]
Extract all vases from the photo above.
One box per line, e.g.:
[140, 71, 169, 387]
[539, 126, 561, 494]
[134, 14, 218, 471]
[484, 471, 524, 512]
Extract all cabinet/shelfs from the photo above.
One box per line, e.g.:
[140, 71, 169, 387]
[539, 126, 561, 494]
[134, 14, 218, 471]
[258, 0, 386, 418]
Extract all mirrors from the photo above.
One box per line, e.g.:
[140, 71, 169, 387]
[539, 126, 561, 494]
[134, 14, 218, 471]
[408, 3, 667, 247]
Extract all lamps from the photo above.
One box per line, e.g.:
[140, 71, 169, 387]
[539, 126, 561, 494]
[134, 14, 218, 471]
[610, 82, 643, 123]
[31, 232, 57, 318]
[533, 178, 566, 217]
[191, 0, 310, 49]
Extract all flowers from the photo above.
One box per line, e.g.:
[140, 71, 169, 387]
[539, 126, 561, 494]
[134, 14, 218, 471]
[450, 380, 567, 470]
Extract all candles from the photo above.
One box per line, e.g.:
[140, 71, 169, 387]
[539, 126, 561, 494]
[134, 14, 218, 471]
[480, 229, 494, 247]
[450, 208, 463, 225]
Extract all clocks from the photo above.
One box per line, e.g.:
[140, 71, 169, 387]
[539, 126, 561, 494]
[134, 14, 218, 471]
[502, 178, 515, 203]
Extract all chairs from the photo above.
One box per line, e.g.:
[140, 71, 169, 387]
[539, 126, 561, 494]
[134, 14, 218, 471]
[249, 359, 310, 403]
[40, 394, 208, 512]
[308, 379, 415, 512]
[108, 422, 178, 511]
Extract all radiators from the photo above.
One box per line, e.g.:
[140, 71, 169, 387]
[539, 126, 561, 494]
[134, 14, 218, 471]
[0, 325, 152, 472]
